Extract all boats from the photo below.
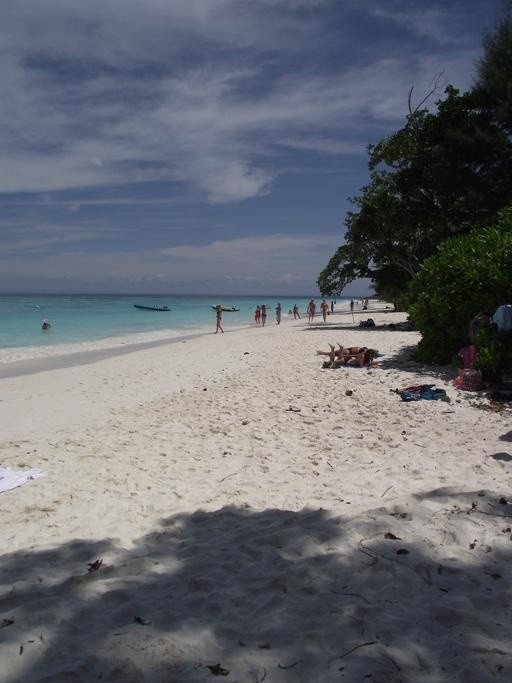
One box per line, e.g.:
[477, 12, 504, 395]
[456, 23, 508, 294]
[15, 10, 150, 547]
[132, 303, 170, 311]
[212, 304, 239, 311]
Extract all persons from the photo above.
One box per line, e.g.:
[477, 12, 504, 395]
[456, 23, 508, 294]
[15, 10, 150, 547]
[349, 297, 370, 311]
[316, 345, 378, 353]
[41, 317, 54, 328]
[253, 297, 334, 328]
[215, 304, 224, 335]
[327, 342, 372, 368]
